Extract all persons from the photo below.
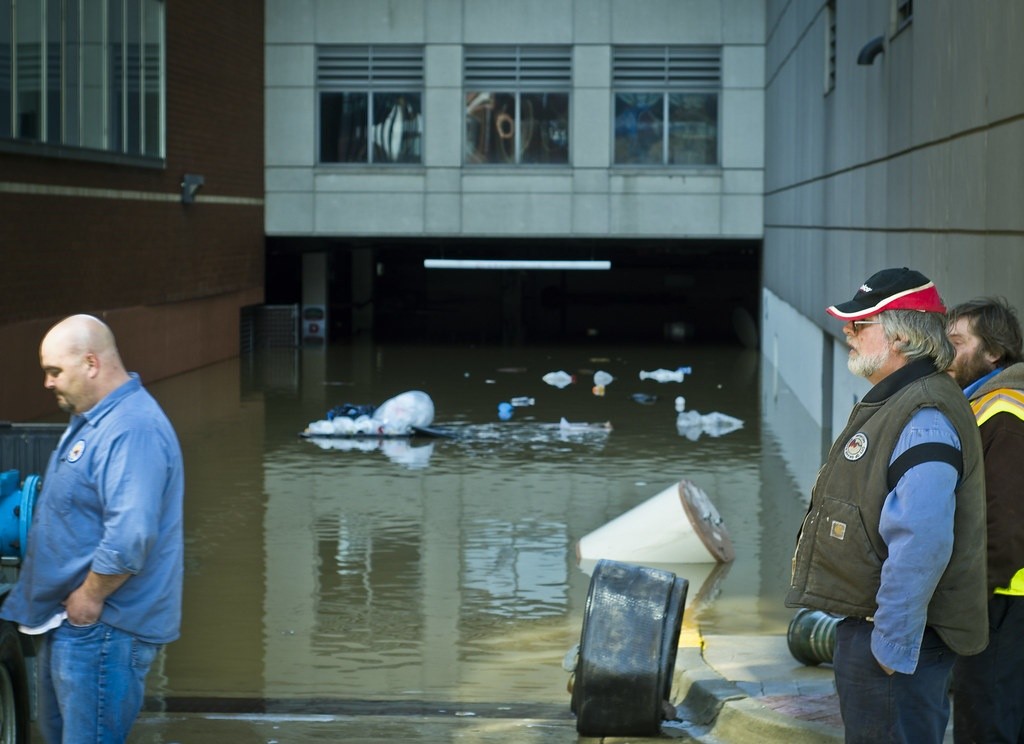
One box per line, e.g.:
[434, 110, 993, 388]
[1, 314, 184, 744]
[785, 266, 1024, 744]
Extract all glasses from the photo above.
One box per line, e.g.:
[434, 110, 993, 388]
[853, 321, 881, 334]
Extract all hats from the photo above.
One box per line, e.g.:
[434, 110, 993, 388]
[826, 266, 946, 320]
